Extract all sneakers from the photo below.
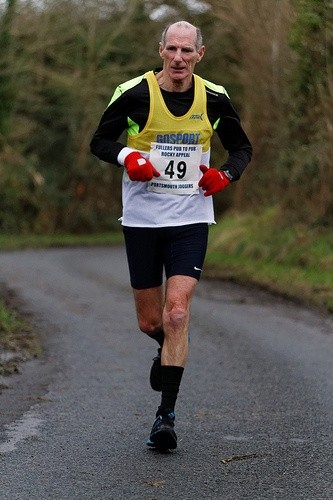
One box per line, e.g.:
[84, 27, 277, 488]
[148, 348, 162, 392]
[146, 414, 178, 450]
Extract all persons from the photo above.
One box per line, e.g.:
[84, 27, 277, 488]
[90, 21, 254, 451]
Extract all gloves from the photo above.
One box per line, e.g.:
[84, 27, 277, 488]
[198, 164, 230, 197]
[124, 151, 160, 182]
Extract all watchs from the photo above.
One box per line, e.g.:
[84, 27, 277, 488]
[218, 169, 233, 181]
